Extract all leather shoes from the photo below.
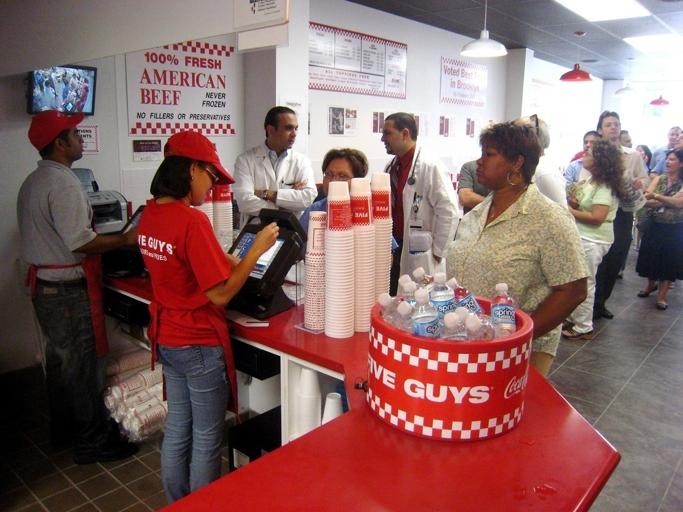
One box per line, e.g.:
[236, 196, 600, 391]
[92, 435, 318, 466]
[603, 308, 613, 319]
[637, 286, 658, 298]
[76, 437, 137, 467]
[656, 298, 668, 310]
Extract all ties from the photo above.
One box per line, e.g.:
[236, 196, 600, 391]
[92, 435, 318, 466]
[391, 160, 400, 208]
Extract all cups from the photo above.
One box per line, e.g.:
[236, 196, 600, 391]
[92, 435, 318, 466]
[302, 171, 394, 338]
[83, 324, 168, 443]
[189, 176, 243, 253]
[297, 366, 321, 431]
[323, 392, 344, 425]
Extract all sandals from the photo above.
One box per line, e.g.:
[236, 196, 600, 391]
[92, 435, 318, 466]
[562, 320, 573, 329]
[562, 328, 593, 340]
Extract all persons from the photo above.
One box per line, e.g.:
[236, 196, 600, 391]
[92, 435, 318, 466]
[636, 144, 651, 177]
[510, 113, 568, 209]
[650, 127, 682, 172]
[620, 130, 633, 149]
[297, 147, 400, 268]
[137, 130, 282, 504]
[557, 137, 630, 341]
[444, 121, 589, 377]
[16, 109, 139, 466]
[637, 146, 682, 312]
[379, 112, 462, 298]
[32, 65, 95, 114]
[563, 130, 601, 194]
[235, 104, 319, 224]
[457, 154, 486, 210]
[649, 131, 683, 181]
[592, 109, 652, 321]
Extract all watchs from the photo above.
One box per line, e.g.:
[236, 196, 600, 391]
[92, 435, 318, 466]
[262, 189, 270, 199]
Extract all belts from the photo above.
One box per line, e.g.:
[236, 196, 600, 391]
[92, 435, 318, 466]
[33, 279, 88, 292]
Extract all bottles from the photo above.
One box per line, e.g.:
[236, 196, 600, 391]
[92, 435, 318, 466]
[456, 306, 468, 318]
[430, 273, 454, 312]
[413, 290, 440, 338]
[492, 282, 516, 337]
[402, 282, 417, 301]
[399, 274, 414, 294]
[377, 294, 399, 314]
[447, 275, 486, 314]
[441, 313, 468, 339]
[465, 313, 495, 340]
[398, 300, 415, 326]
[412, 267, 432, 286]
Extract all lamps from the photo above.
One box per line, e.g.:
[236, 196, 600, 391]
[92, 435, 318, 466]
[561, 31, 591, 83]
[649, 72, 671, 103]
[458, 1, 508, 61]
[615, 61, 639, 97]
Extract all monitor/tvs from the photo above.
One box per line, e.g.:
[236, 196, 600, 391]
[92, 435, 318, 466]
[28, 63, 98, 115]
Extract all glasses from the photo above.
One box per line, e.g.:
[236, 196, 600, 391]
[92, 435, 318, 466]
[321, 171, 347, 182]
[530, 111, 539, 134]
[204, 168, 219, 184]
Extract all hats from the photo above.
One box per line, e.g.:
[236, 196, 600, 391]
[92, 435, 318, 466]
[27, 111, 86, 150]
[164, 130, 234, 186]
